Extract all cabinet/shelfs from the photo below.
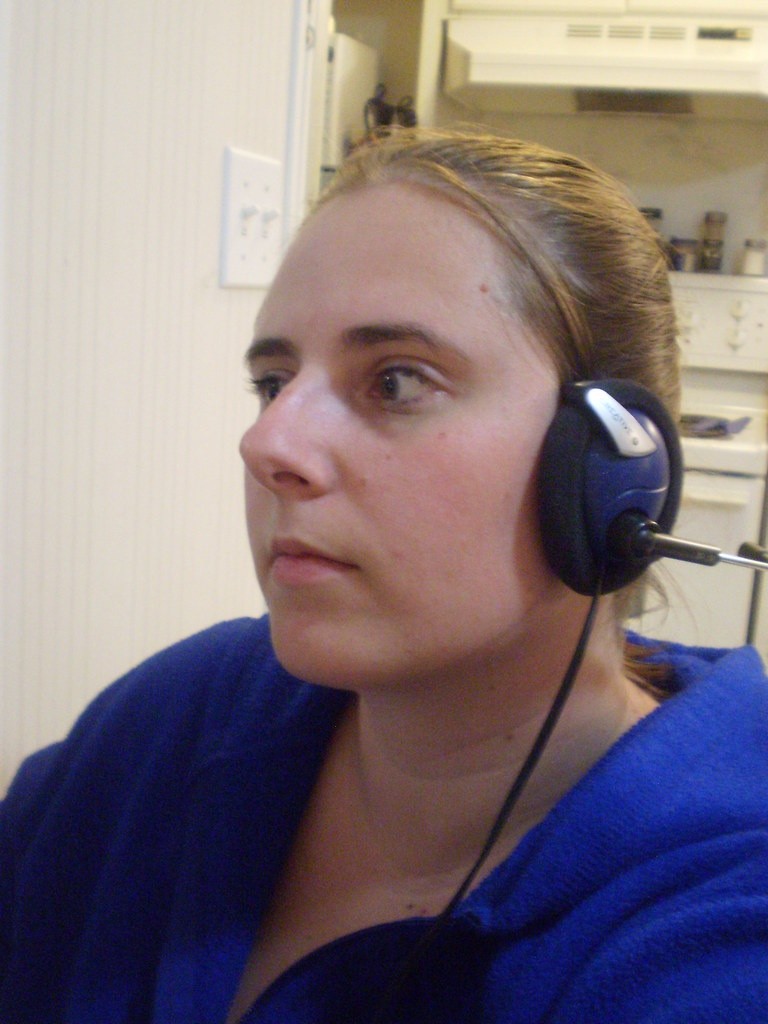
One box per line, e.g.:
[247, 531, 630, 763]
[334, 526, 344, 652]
[416, 0, 768, 668]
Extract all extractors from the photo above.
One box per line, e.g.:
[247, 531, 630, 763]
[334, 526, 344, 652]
[442, 17, 768, 118]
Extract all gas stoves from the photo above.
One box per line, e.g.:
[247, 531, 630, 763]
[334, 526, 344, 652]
[665, 272, 768, 476]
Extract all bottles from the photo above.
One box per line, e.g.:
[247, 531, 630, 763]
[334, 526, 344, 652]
[701, 211, 727, 272]
[742, 238, 767, 276]
[670, 238, 698, 271]
[639, 207, 661, 233]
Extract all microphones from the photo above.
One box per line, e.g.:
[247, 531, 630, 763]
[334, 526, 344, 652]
[610, 511, 768, 572]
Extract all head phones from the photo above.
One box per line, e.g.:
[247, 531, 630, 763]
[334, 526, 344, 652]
[534, 376, 684, 596]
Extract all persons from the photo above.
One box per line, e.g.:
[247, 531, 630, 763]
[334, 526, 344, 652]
[0, 122, 768, 1023]
